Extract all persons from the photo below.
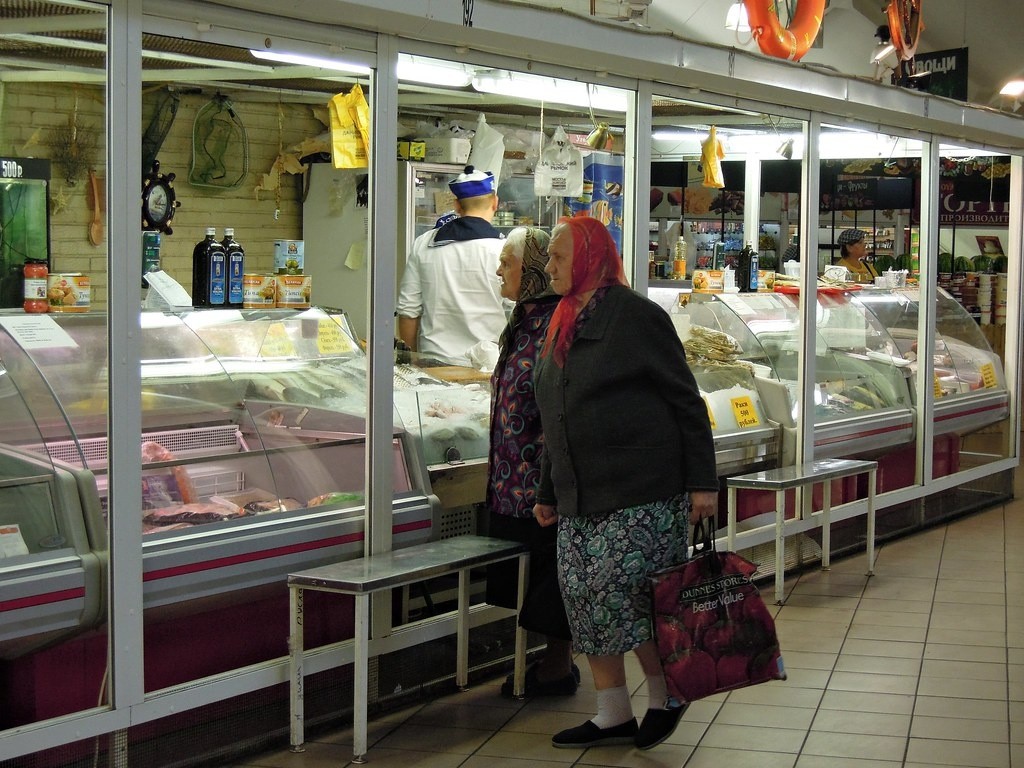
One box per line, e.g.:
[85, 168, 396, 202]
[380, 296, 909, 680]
[822, 226, 878, 283]
[394, 164, 513, 355]
[478, 225, 583, 698]
[534, 214, 724, 752]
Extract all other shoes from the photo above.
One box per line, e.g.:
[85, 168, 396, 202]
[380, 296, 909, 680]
[552, 717, 639, 748]
[634, 701, 691, 750]
[502, 658, 583, 698]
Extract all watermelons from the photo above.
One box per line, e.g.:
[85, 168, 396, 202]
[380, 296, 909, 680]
[872, 251, 1008, 276]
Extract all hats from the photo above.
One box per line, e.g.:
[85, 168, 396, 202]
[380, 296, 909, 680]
[837, 228, 868, 245]
[781, 245, 797, 263]
[448, 165, 494, 199]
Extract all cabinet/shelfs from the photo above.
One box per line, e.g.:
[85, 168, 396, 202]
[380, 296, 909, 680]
[3, 273, 1024, 759]
[687, 222, 779, 270]
[860, 226, 895, 265]
[296, 165, 559, 352]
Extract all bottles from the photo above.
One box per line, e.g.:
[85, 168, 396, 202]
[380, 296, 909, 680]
[219, 227, 244, 309]
[737, 240, 758, 292]
[192, 227, 225, 309]
[673, 235, 687, 280]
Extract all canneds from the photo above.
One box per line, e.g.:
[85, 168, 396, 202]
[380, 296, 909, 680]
[938, 272, 1007, 326]
[242, 239, 312, 311]
[690, 270, 725, 293]
[23, 258, 93, 314]
[651, 260, 665, 279]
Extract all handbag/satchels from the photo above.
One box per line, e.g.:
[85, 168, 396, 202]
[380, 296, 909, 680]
[648, 515, 789, 701]
